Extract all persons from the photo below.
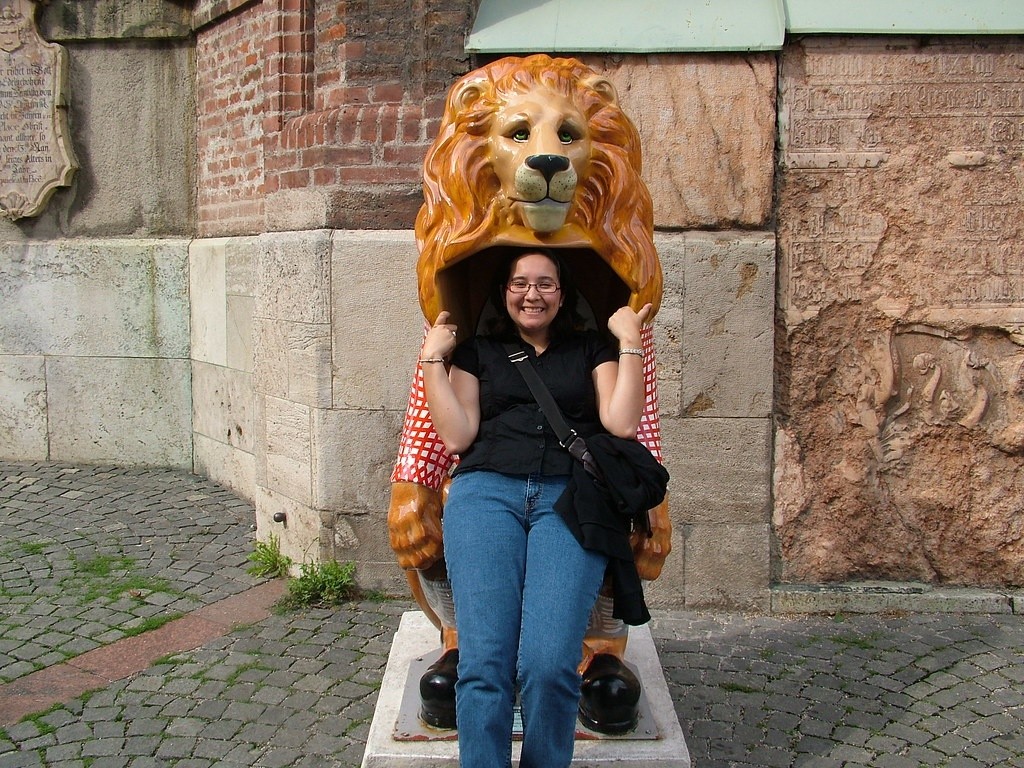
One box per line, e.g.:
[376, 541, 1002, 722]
[414, 243, 654, 768]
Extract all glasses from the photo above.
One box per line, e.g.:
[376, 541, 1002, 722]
[505, 281, 561, 293]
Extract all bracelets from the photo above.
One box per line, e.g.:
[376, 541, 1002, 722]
[419, 358, 444, 363]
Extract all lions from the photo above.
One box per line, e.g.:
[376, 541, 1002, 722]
[386, 52, 673, 735]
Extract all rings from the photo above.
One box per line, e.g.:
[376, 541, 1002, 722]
[452, 331, 456, 337]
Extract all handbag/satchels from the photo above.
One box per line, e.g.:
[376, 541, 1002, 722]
[568, 437, 604, 484]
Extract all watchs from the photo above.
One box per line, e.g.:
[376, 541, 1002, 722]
[619, 348, 645, 358]
